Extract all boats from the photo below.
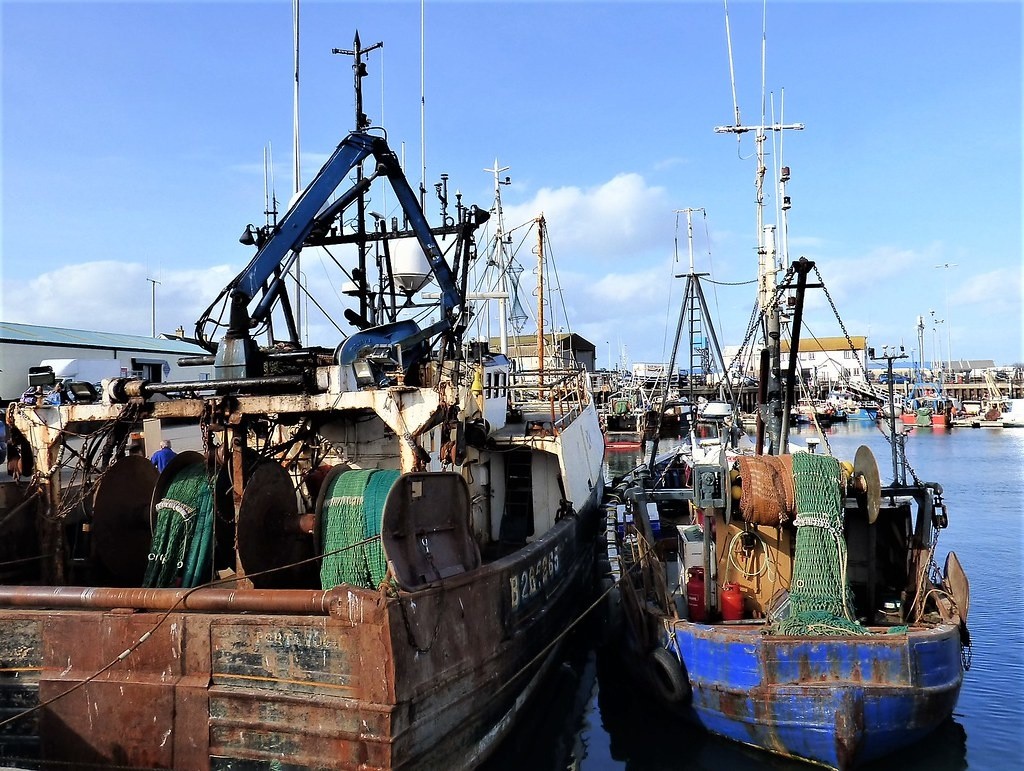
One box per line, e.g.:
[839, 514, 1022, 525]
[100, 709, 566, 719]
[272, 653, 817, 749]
[0, 0, 628, 771]
[588, 308, 1024, 434]
[604, 0, 974, 770]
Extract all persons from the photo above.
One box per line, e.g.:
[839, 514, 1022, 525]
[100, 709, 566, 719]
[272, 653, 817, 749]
[150, 439, 177, 474]
